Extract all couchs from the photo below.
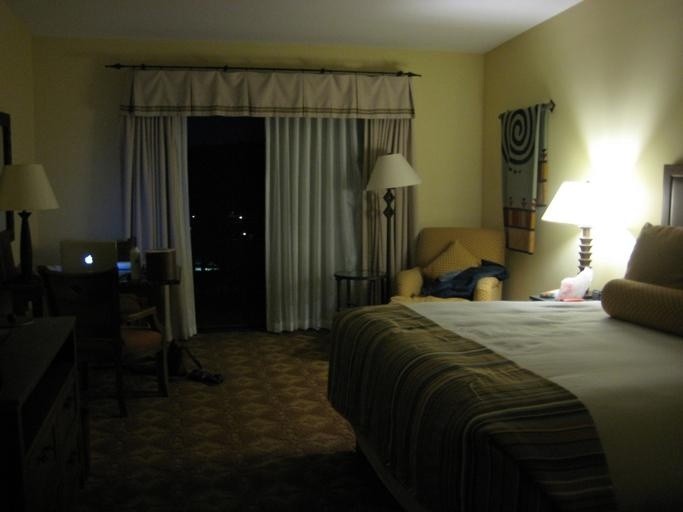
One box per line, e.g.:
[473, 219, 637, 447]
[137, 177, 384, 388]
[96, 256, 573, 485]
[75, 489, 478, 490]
[389, 227, 507, 303]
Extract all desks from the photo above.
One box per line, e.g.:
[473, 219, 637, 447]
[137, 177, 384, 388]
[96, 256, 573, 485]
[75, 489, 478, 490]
[38, 262, 183, 287]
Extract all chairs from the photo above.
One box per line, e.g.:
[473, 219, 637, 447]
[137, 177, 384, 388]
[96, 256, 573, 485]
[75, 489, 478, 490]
[38, 265, 166, 419]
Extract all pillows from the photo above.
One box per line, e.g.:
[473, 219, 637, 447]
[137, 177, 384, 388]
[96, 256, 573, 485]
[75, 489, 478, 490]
[599, 278, 682, 335]
[624, 222, 683, 286]
[422, 240, 482, 281]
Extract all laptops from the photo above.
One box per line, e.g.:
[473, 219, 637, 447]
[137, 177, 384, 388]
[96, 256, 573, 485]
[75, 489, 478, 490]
[62, 239, 117, 279]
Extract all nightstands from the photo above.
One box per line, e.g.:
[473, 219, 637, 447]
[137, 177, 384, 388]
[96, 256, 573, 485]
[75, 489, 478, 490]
[529, 291, 602, 301]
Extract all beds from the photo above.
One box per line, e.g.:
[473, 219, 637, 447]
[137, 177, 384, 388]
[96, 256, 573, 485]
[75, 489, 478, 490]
[325, 164, 683, 511]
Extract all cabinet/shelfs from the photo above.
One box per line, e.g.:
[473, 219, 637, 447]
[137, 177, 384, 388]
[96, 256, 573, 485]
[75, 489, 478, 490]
[0, 315, 88, 512]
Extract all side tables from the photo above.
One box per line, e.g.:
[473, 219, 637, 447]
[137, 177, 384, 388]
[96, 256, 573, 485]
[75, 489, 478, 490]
[333, 269, 385, 309]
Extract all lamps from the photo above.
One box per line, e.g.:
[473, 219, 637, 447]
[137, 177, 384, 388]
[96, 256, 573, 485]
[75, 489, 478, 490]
[0, 162, 60, 316]
[366, 153, 423, 305]
[540, 181, 594, 276]
